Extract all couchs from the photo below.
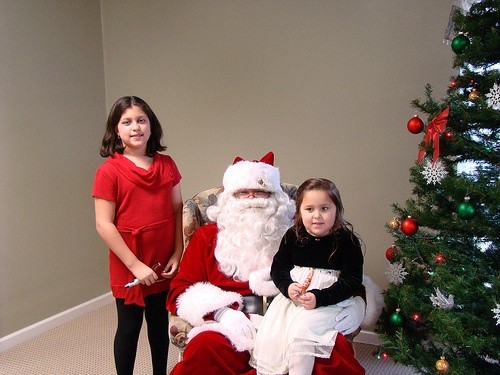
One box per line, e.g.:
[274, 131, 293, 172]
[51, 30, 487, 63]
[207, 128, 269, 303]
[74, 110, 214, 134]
[168, 183, 362, 356]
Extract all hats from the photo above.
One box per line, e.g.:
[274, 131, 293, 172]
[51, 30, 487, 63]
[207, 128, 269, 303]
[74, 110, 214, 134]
[223, 151, 281, 195]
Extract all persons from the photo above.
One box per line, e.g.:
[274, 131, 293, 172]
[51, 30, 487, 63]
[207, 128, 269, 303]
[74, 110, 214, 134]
[91, 96, 184, 375]
[167, 151, 370, 375]
[254, 177, 368, 375]
